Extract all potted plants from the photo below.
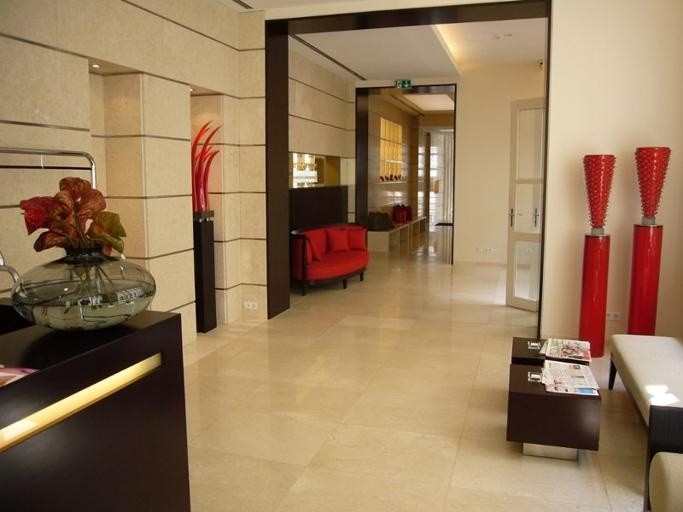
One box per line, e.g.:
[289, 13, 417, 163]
[9, 177, 157, 332]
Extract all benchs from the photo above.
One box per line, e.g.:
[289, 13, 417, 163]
[607, 334, 683, 512]
[368, 216, 428, 253]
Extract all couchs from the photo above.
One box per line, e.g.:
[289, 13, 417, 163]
[291, 221, 369, 296]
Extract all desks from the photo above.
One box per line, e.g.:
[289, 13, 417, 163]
[506, 336, 600, 461]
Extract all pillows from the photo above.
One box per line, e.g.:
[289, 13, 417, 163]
[309, 228, 367, 262]
